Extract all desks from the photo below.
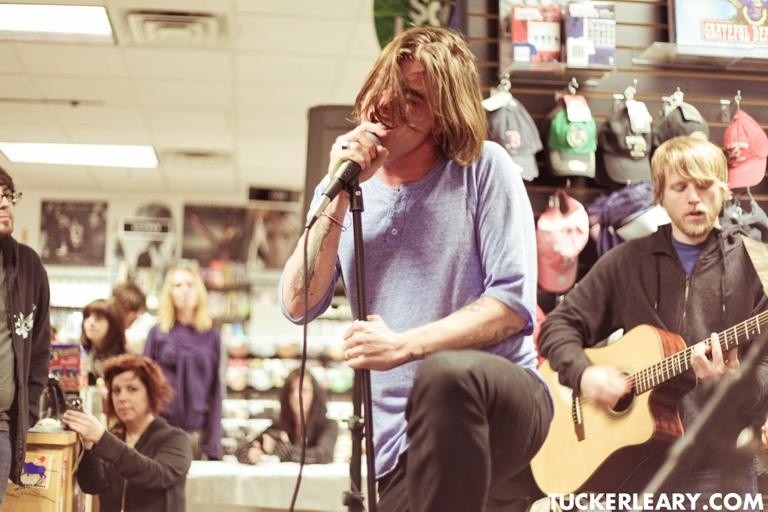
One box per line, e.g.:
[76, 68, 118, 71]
[23, 417, 121, 512]
[178, 459, 369, 511]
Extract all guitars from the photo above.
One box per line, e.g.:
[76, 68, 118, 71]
[529, 310, 768, 498]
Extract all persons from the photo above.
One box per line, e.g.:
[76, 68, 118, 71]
[257, 209, 306, 271]
[236, 367, 342, 466]
[278, 25, 554, 511]
[61, 258, 227, 512]
[1, 163, 50, 511]
[40, 200, 108, 264]
[537, 135, 767, 510]
[191, 209, 259, 262]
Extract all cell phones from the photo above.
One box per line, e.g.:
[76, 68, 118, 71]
[66, 397, 83, 431]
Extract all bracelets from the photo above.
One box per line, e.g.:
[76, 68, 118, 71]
[316, 211, 345, 230]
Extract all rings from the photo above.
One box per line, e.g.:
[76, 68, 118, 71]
[341, 142, 350, 151]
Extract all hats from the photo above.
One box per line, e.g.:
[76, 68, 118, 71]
[600, 107, 653, 183]
[485, 97, 543, 182]
[722, 110, 768, 189]
[653, 107, 710, 148]
[545, 106, 598, 179]
[537, 189, 589, 293]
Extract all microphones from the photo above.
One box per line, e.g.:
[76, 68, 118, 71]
[305, 131, 382, 230]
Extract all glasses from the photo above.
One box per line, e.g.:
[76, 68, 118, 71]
[0, 191, 24, 205]
